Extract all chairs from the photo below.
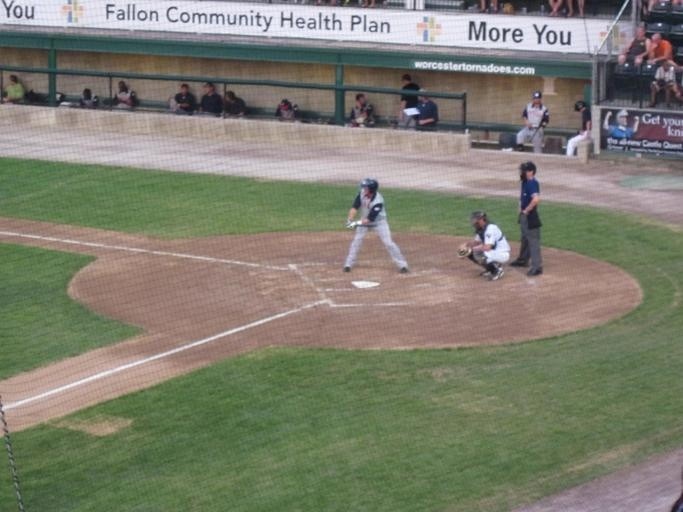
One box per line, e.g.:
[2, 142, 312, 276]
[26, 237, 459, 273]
[611, 0, 681, 81]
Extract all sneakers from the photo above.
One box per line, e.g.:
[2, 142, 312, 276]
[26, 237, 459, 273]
[481, 268, 492, 277]
[516, 146, 524, 151]
[510, 257, 529, 267]
[491, 267, 505, 281]
[527, 267, 543, 275]
[400, 267, 408, 274]
[343, 265, 351, 272]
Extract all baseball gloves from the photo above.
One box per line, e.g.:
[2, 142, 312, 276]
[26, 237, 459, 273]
[455, 242, 471, 258]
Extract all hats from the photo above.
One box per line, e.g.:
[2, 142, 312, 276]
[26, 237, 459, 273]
[533, 92, 541, 100]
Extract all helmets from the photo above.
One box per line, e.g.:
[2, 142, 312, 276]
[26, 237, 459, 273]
[616, 111, 629, 120]
[471, 211, 487, 229]
[360, 179, 378, 194]
[519, 161, 537, 177]
[575, 100, 586, 111]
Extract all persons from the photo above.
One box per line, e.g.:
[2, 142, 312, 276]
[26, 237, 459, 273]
[3, 75, 24, 104]
[465, 211, 512, 281]
[616, 0, 682, 106]
[112, 80, 136, 109]
[294, 0, 388, 8]
[199, 82, 223, 117]
[169, 84, 194, 115]
[603, 110, 639, 141]
[566, 102, 592, 156]
[549, 1, 584, 17]
[344, 178, 408, 274]
[274, 99, 296, 123]
[222, 91, 245, 117]
[508, 162, 543, 276]
[479, 0, 498, 13]
[515, 91, 549, 153]
[351, 94, 376, 128]
[80, 89, 99, 108]
[413, 89, 439, 132]
[398, 74, 422, 129]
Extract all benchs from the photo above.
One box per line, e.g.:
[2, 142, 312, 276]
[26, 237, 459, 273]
[25, 92, 567, 160]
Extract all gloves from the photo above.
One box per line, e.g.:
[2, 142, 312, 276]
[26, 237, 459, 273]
[345, 219, 356, 229]
